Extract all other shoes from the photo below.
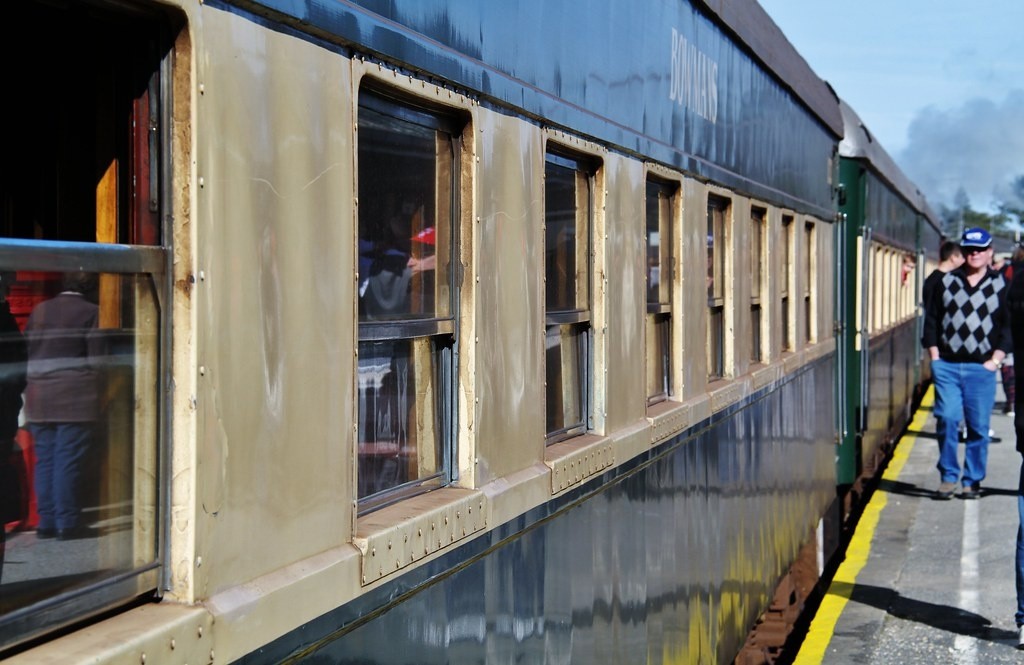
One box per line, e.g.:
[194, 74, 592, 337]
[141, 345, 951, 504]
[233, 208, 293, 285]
[961, 484, 979, 499]
[1007, 411, 1015, 419]
[35, 527, 59, 539]
[938, 481, 960, 500]
[962, 427, 994, 439]
[56, 523, 99, 541]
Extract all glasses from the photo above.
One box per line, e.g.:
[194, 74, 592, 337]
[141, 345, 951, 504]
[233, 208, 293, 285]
[963, 246, 991, 254]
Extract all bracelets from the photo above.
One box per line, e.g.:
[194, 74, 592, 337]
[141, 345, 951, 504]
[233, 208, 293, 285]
[991, 357, 1002, 368]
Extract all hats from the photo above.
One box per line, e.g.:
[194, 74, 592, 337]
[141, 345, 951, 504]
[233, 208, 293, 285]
[409, 226, 436, 245]
[959, 228, 993, 247]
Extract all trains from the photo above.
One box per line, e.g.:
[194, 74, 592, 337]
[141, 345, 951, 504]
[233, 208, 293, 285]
[0, 1, 949, 665]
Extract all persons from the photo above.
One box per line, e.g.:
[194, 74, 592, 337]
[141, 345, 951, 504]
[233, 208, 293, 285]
[360, 244, 411, 319]
[920, 227, 1024, 649]
[0, 267, 113, 565]
